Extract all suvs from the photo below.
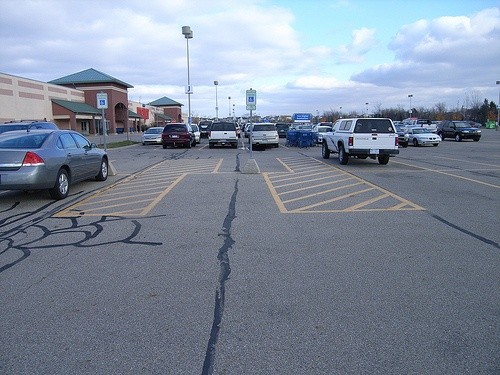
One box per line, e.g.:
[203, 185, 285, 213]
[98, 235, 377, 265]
[436, 120, 482, 142]
[161, 122, 195, 149]
[322, 118, 399, 164]
[0, 119, 59, 135]
[247, 122, 279, 150]
[208, 121, 238, 149]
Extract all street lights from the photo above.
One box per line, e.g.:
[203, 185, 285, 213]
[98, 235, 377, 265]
[408, 95, 413, 119]
[182, 25, 193, 126]
[228, 96, 231, 117]
[213, 80, 219, 122]
[339, 107, 342, 119]
[496, 80, 500, 126]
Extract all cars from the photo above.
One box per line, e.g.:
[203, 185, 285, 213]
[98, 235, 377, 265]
[393, 118, 443, 134]
[198, 120, 213, 139]
[234, 121, 251, 138]
[394, 124, 410, 148]
[141, 126, 164, 146]
[190, 124, 201, 144]
[0, 129, 109, 201]
[274, 121, 333, 149]
[408, 127, 442, 147]
